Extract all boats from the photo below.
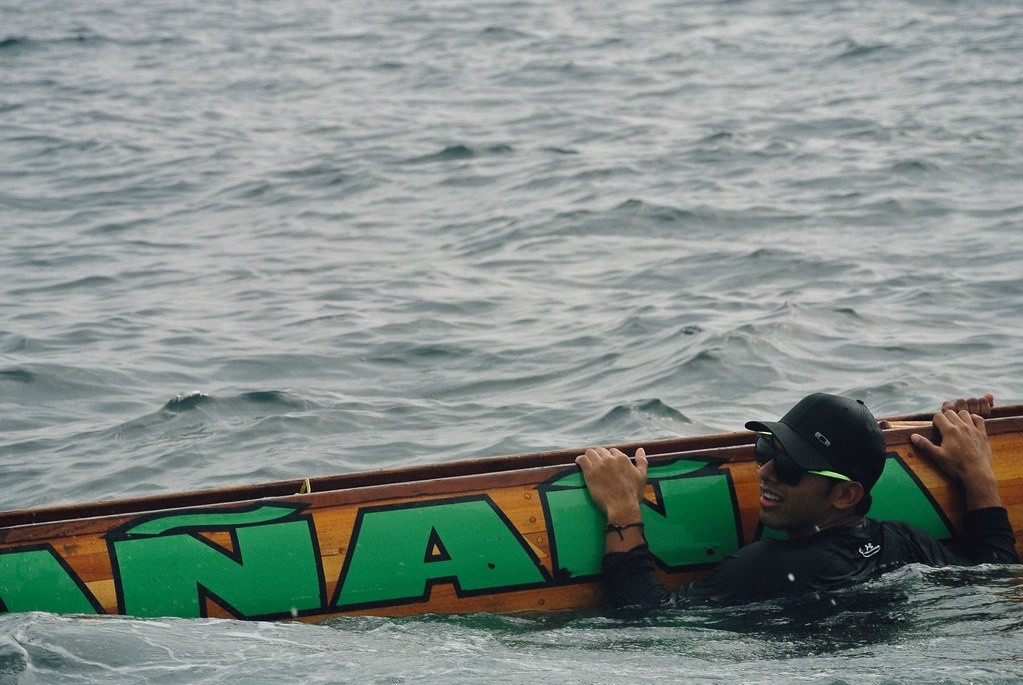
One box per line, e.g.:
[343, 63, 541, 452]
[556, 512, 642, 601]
[0, 403, 1022, 626]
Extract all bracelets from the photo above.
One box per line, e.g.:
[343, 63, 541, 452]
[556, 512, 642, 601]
[604, 522, 645, 540]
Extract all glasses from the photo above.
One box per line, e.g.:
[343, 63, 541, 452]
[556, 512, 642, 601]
[753, 435, 852, 486]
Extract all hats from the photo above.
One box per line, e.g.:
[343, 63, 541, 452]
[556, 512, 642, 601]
[746, 393, 886, 496]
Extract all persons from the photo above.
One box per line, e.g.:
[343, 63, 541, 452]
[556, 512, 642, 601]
[576, 392, 1017, 613]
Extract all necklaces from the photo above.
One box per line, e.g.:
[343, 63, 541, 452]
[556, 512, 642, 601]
[790, 512, 855, 537]
